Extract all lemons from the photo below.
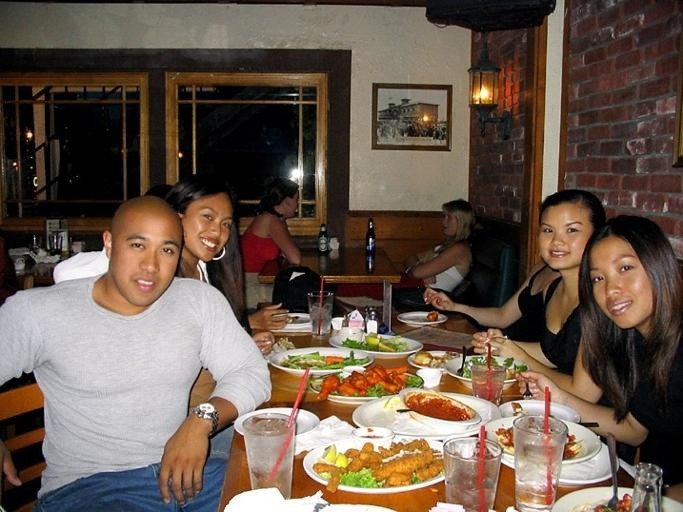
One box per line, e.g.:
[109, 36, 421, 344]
[365, 332, 381, 346]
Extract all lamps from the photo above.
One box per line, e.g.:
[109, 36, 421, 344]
[469, 39, 512, 140]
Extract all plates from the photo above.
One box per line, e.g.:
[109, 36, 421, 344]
[234, 407, 320, 441]
[407, 351, 465, 372]
[331, 317, 344, 329]
[272, 313, 313, 329]
[397, 312, 448, 329]
[303, 427, 444, 493]
[352, 387, 499, 442]
[270, 347, 375, 379]
[482, 399, 621, 488]
[549, 486, 683, 511]
[309, 365, 424, 405]
[329, 333, 423, 359]
[444, 355, 531, 391]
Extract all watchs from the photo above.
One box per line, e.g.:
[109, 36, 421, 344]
[190, 400, 219, 440]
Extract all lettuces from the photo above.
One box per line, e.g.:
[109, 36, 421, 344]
[319, 464, 419, 490]
[281, 336, 422, 399]
[459, 356, 527, 380]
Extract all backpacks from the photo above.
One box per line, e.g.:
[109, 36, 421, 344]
[273, 265, 356, 318]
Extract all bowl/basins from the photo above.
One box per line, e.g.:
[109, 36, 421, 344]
[352, 426, 395, 448]
[416, 368, 443, 388]
[397, 387, 481, 432]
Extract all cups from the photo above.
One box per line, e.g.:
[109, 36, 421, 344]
[243, 411, 295, 501]
[470, 364, 507, 405]
[444, 439, 503, 512]
[512, 415, 567, 512]
[307, 291, 332, 341]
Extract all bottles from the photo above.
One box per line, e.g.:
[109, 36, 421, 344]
[318, 256, 326, 269]
[330, 236, 339, 249]
[366, 258, 376, 277]
[363, 304, 380, 333]
[30, 233, 63, 261]
[317, 224, 329, 254]
[329, 249, 339, 260]
[628, 462, 662, 512]
[365, 218, 376, 258]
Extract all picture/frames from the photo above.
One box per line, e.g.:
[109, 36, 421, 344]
[371, 82, 452, 151]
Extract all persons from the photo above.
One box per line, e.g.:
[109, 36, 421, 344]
[517, 217, 682, 503]
[470, 189, 614, 407]
[0, 195, 273, 512]
[50, 177, 303, 359]
[420, 255, 562, 340]
[333, 200, 474, 311]
